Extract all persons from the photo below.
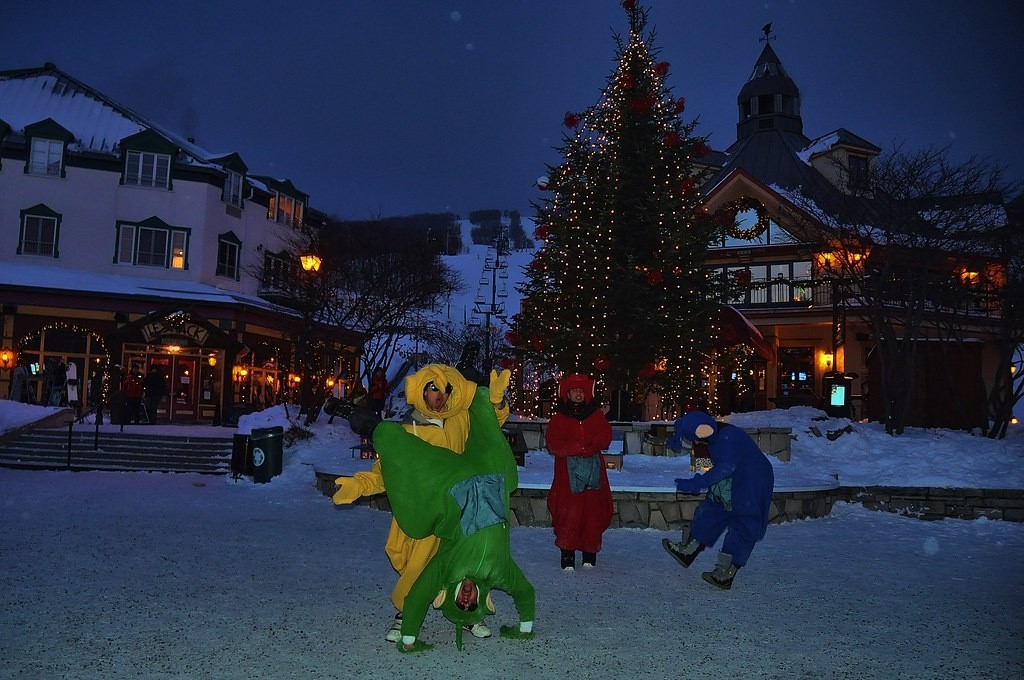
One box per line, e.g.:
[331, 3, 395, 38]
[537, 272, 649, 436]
[547, 374, 613, 571]
[663, 411, 774, 590]
[122, 362, 165, 424]
[332, 364, 512, 642]
[323, 342, 536, 651]
[370, 367, 390, 412]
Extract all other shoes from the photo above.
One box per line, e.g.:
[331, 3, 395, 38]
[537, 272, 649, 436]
[561, 551, 576, 571]
[582, 552, 596, 568]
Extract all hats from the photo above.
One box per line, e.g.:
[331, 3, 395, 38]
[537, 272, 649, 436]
[374, 367, 382, 374]
[150, 365, 159, 371]
[132, 362, 139, 369]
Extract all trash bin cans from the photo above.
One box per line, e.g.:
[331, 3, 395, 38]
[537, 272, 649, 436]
[231, 433, 254, 475]
[250, 426, 284, 484]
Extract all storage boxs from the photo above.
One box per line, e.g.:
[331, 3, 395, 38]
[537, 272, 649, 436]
[604, 455, 623, 472]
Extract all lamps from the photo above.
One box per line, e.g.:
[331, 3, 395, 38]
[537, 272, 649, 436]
[824, 248, 832, 266]
[3, 347, 10, 366]
[825, 348, 833, 366]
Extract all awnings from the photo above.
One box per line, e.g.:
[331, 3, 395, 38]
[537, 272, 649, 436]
[719, 305, 775, 365]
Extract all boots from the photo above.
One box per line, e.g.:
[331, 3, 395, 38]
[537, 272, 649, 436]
[663, 536, 706, 568]
[702, 549, 741, 590]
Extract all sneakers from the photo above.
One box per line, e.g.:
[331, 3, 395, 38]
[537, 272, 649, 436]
[386, 611, 405, 643]
[462, 618, 491, 638]
[455, 341, 480, 371]
[323, 397, 358, 421]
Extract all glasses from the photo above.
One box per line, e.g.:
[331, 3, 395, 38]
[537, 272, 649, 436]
[429, 384, 453, 394]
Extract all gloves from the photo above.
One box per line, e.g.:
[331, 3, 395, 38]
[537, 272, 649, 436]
[673, 474, 707, 497]
[488, 368, 511, 404]
[498, 622, 535, 641]
[396, 636, 433, 653]
[576, 440, 598, 458]
[333, 476, 366, 505]
